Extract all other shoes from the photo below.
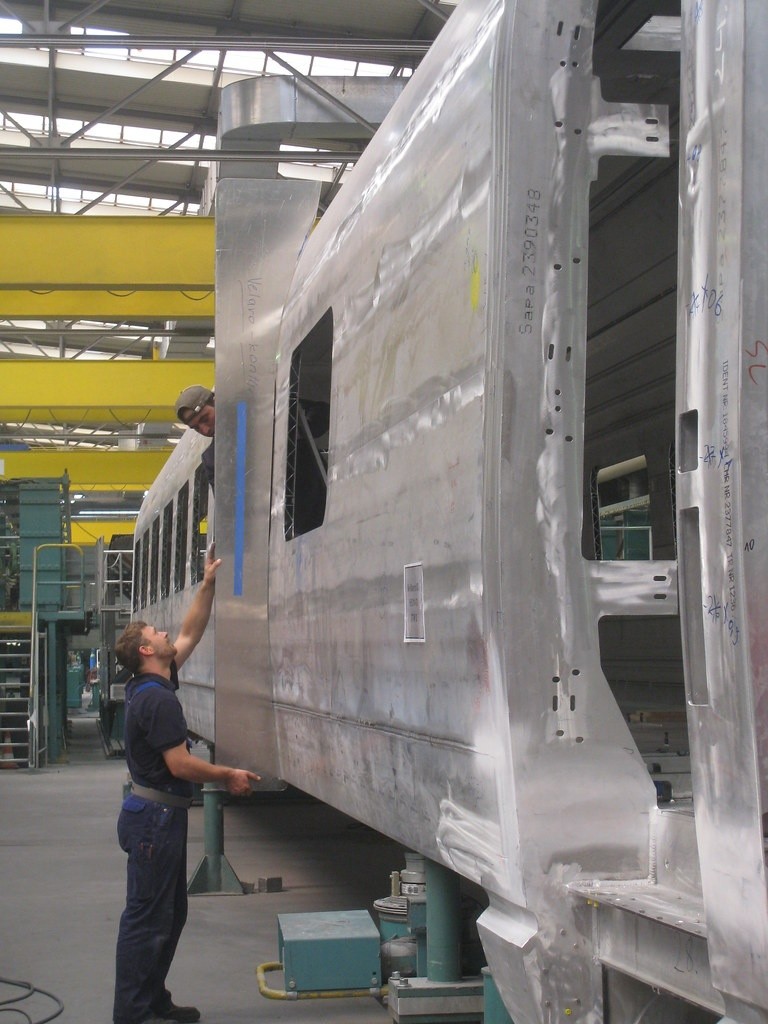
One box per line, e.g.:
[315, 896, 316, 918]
[139, 1014, 179, 1024]
[154, 1000, 201, 1023]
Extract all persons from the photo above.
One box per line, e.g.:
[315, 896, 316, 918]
[174, 385, 216, 492]
[115, 541, 263, 1024]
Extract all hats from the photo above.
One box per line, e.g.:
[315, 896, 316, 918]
[175, 385, 213, 424]
